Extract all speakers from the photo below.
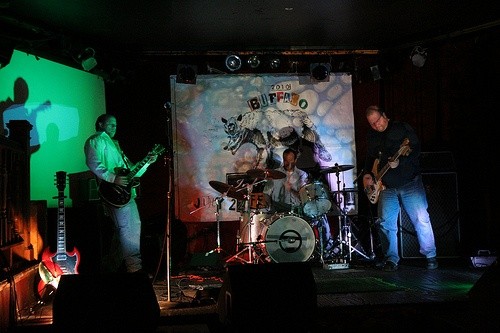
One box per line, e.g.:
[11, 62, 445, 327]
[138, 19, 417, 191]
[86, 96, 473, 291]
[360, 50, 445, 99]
[215, 261, 318, 326]
[52, 270, 161, 333]
[397, 172, 461, 260]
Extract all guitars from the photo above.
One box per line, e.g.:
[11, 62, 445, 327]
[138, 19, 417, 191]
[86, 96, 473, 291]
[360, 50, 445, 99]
[362, 138, 409, 204]
[97, 144, 166, 209]
[36, 170, 82, 302]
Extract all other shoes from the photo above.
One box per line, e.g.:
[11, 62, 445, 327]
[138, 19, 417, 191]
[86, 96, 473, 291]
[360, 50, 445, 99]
[425, 256, 439, 270]
[383, 261, 398, 272]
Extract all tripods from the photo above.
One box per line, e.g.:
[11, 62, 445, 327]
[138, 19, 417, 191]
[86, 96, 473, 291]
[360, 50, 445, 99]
[310, 168, 375, 267]
[190, 172, 268, 266]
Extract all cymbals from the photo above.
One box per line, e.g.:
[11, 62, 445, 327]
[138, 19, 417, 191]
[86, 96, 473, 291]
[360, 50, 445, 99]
[209, 180, 246, 201]
[245, 169, 287, 180]
[320, 165, 356, 173]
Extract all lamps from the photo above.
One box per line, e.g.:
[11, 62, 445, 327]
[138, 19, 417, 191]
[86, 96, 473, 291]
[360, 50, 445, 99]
[226, 55, 330, 82]
[74, 48, 97, 71]
[410, 40, 427, 67]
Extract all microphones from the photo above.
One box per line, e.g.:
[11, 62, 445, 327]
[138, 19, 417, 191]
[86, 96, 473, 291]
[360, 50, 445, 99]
[288, 236, 296, 243]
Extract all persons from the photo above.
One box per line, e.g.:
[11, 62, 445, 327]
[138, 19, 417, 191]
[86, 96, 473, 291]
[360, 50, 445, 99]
[363, 105, 437, 269]
[263, 148, 308, 205]
[84, 114, 158, 272]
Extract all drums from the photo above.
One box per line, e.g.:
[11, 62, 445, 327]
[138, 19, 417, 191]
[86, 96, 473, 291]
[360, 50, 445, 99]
[240, 182, 333, 263]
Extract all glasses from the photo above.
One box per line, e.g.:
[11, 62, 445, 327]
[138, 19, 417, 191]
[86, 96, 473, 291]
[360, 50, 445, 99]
[370, 115, 382, 127]
